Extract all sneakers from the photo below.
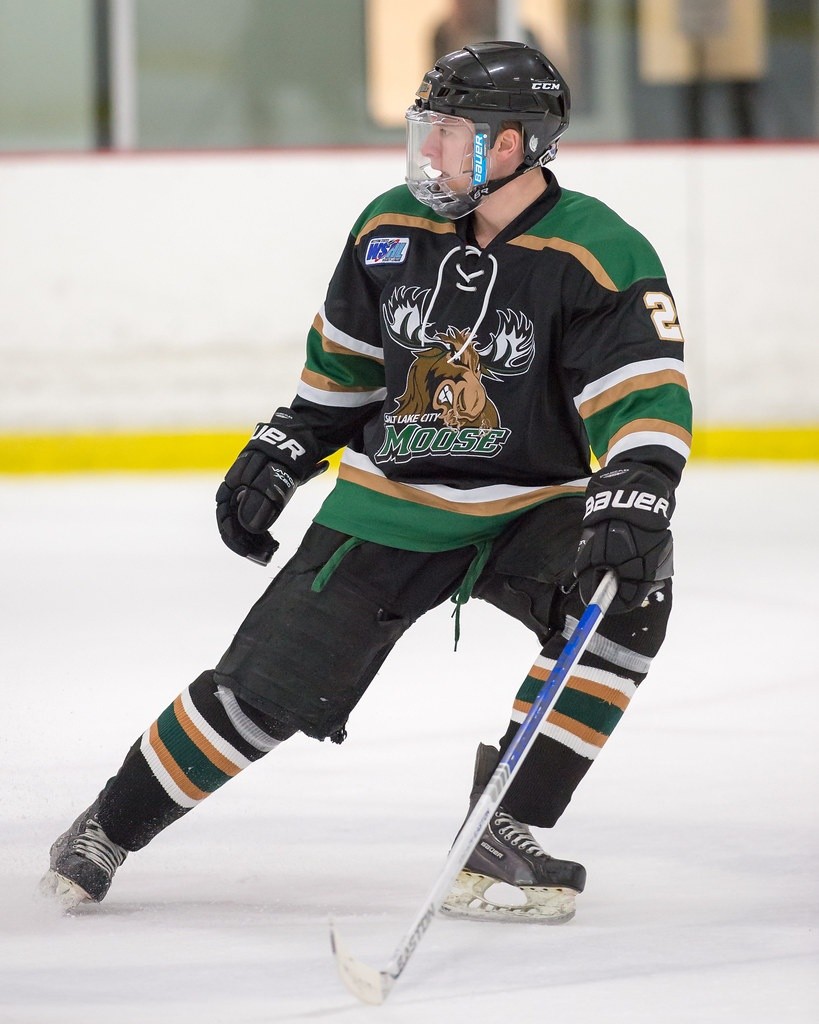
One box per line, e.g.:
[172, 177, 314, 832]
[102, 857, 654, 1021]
[37, 776, 129, 915]
[433, 742, 586, 927]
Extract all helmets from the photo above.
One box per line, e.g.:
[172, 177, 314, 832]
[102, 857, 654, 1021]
[404, 41, 571, 220]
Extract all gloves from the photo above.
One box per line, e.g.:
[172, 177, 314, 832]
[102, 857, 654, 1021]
[576, 461, 674, 615]
[215, 406, 330, 568]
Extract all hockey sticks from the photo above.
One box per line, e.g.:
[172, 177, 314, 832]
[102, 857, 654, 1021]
[330, 573, 618, 1009]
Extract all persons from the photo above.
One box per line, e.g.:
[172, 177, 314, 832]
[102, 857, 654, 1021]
[432, 0, 541, 70]
[40, 41, 695, 922]
[627, 0, 773, 140]
